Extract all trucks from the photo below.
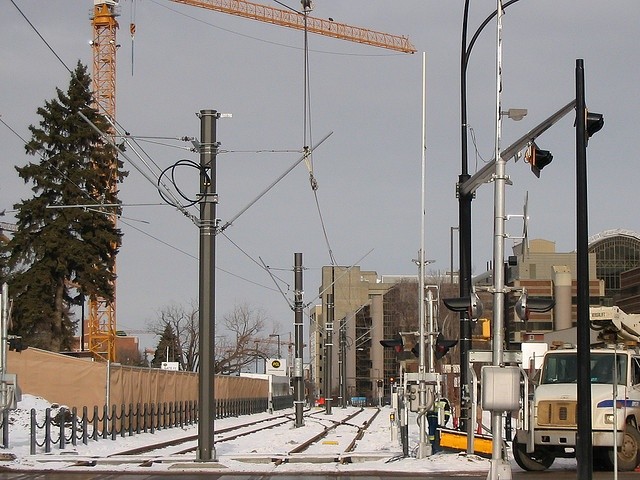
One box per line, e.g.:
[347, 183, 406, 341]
[511, 305, 639, 472]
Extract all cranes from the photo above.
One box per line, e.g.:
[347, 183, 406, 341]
[85, 1, 419, 361]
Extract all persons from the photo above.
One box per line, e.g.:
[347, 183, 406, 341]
[428, 397, 451, 447]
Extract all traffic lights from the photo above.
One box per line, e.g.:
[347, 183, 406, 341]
[433, 332, 458, 360]
[379, 333, 406, 355]
[585, 108, 604, 137]
[515, 293, 557, 325]
[532, 143, 553, 178]
[442, 293, 484, 319]
